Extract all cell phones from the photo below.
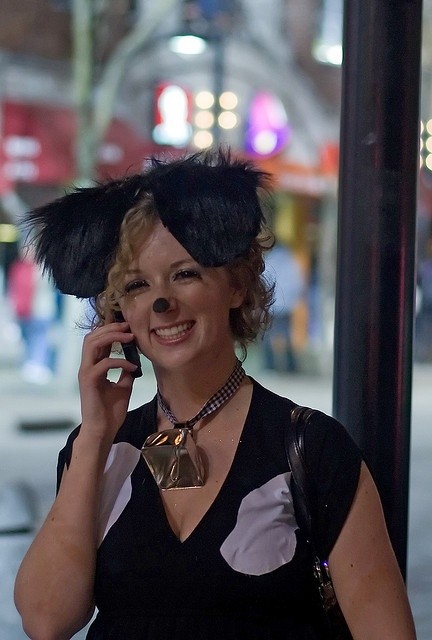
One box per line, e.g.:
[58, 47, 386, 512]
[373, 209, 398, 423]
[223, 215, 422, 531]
[114, 303, 143, 378]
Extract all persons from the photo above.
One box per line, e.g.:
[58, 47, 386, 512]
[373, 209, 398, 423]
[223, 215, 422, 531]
[9, 240, 38, 349]
[254, 228, 303, 372]
[20, 261, 65, 391]
[14, 145, 416, 640]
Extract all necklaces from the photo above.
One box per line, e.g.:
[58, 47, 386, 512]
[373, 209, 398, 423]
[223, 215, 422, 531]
[139, 357, 247, 492]
